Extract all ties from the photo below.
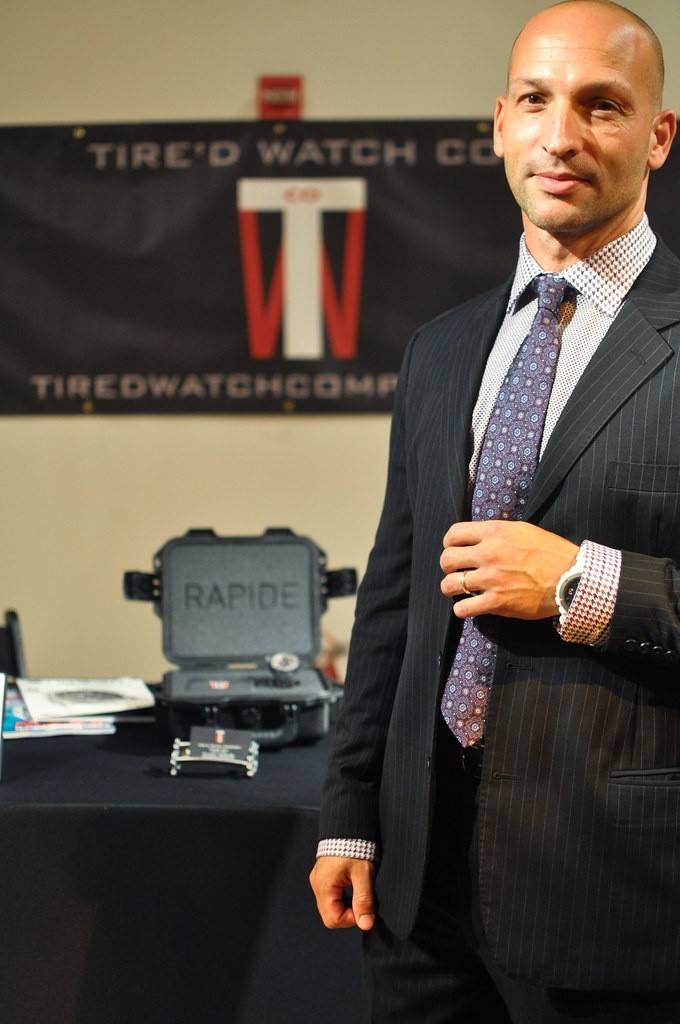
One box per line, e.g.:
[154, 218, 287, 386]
[440, 275, 577, 747]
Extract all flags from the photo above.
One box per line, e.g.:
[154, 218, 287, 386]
[1, 116, 680, 415]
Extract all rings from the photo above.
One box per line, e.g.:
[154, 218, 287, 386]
[461, 570, 474, 595]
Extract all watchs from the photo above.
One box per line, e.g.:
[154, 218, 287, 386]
[555, 545, 585, 626]
[265, 651, 302, 688]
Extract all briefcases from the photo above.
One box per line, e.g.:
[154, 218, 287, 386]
[154, 527, 330, 748]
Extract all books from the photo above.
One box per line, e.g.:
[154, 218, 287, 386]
[3, 675, 156, 740]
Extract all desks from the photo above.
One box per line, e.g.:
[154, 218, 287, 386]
[1, 682, 372, 1021]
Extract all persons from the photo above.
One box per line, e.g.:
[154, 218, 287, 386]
[309, 1, 679, 1024]
